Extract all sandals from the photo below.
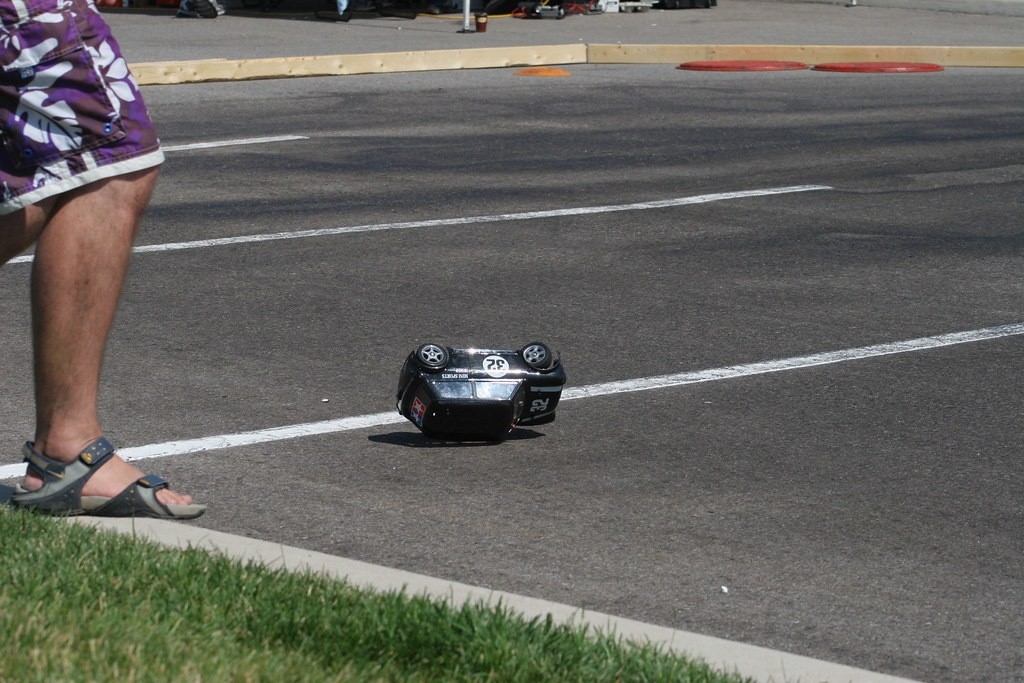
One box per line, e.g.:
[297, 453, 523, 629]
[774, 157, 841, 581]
[11, 436, 207, 519]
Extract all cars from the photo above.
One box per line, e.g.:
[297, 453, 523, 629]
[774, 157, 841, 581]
[396, 342, 566, 443]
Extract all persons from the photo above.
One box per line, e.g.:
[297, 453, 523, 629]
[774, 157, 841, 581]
[0, 0, 205, 522]
